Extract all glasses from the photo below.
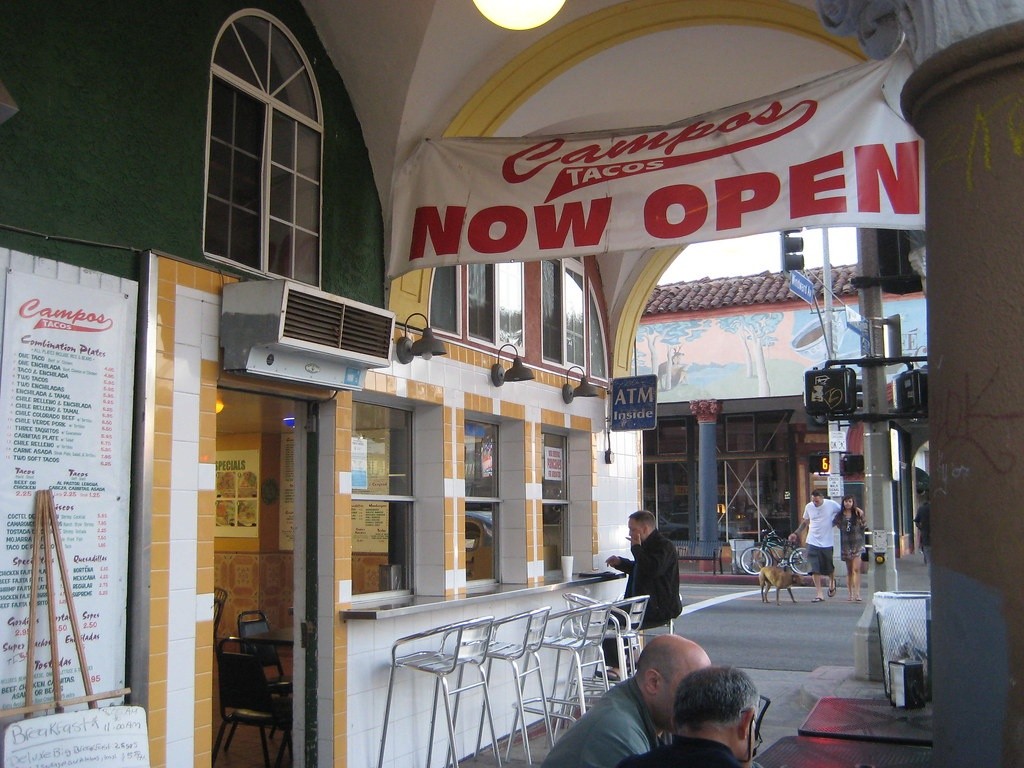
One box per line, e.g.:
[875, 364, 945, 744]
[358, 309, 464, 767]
[846, 521, 851, 532]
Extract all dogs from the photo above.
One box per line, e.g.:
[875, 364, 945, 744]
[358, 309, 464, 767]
[758, 565, 805, 605]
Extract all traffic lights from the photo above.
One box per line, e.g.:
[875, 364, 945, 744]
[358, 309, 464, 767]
[810, 454, 829, 474]
[781, 230, 804, 274]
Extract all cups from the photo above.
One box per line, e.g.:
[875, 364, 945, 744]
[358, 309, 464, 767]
[561, 555, 573, 576]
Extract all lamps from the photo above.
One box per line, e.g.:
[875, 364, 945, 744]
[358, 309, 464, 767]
[563, 366, 599, 404]
[396, 313, 446, 364]
[491, 344, 535, 387]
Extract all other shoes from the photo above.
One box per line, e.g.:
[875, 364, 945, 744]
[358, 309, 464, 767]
[856, 596, 862, 601]
[848, 595, 854, 602]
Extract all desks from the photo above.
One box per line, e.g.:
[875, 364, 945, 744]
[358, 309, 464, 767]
[249, 627, 293, 683]
[750, 695, 933, 768]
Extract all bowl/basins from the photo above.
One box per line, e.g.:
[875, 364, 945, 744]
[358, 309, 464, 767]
[792, 313, 845, 360]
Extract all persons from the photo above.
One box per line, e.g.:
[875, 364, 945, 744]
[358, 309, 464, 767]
[912, 495, 930, 568]
[592, 510, 683, 679]
[806, 493, 872, 601]
[540, 634, 712, 768]
[618, 666, 764, 768]
[787, 489, 864, 603]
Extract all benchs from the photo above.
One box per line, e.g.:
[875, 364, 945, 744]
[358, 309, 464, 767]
[672, 540, 724, 574]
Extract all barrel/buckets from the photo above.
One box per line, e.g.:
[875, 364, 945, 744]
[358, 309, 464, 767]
[729, 539, 754, 574]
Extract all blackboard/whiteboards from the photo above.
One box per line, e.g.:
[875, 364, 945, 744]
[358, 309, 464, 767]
[2, 706, 150, 768]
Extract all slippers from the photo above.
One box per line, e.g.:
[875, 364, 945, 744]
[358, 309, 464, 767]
[811, 596, 825, 603]
[596, 669, 622, 681]
[626, 661, 637, 675]
[828, 578, 836, 597]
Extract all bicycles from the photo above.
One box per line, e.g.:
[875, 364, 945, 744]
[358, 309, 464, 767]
[740, 528, 808, 576]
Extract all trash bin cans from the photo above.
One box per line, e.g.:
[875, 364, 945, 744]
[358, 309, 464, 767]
[729, 538, 755, 575]
[874, 590, 933, 701]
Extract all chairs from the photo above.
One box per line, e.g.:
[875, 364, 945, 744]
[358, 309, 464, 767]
[238, 610, 293, 738]
[379, 593, 682, 768]
[211, 637, 294, 767]
